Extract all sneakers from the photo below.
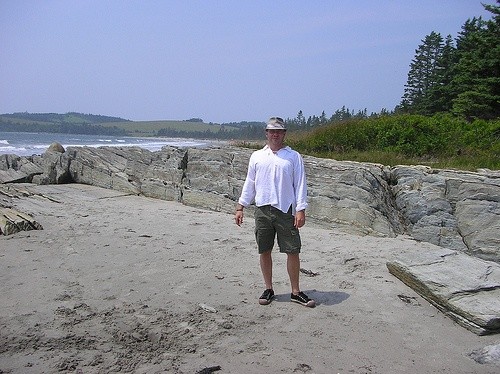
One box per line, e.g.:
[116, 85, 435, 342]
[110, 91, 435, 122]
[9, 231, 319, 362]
[259, 289, 274, 305]
[291, 291, 315, 306]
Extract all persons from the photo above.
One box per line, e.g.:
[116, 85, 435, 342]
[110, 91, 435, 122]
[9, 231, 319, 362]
[235, 117, 315, 309]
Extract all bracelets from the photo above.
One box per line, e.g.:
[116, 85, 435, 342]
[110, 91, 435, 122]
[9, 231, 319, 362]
[236, 208, 243, 212]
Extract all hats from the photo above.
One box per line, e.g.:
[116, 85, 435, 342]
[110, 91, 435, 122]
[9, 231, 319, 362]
[265, 116, 287, 130]
